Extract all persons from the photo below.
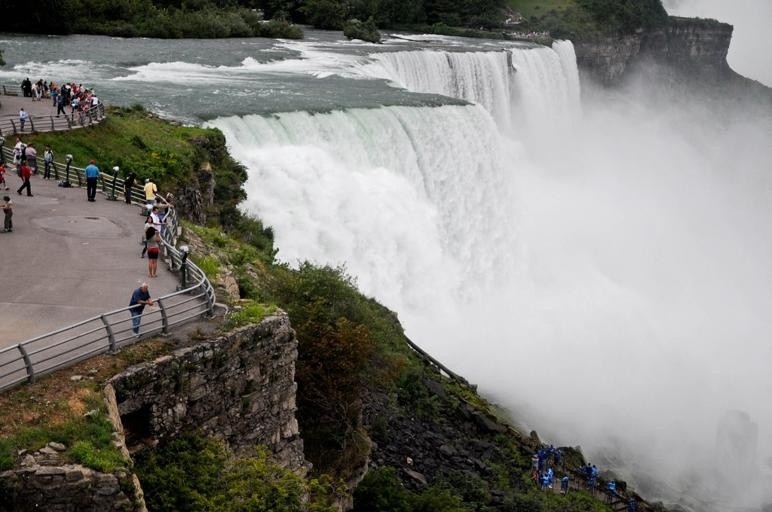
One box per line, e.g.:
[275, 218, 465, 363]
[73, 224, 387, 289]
[129, 282, 154, 339]
[0, 77, 176, 277]
[530, 444, 639, 512]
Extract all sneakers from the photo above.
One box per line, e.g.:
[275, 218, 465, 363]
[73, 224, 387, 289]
[141, 254, 144, 258]
[87, 198, 95, 202]
[0, 229, 13, 233]
[132, 332, 141, 339]
[147, 273, 158, 278]
[27, 194, 33, 197]
[17, 190, 22, 195]
